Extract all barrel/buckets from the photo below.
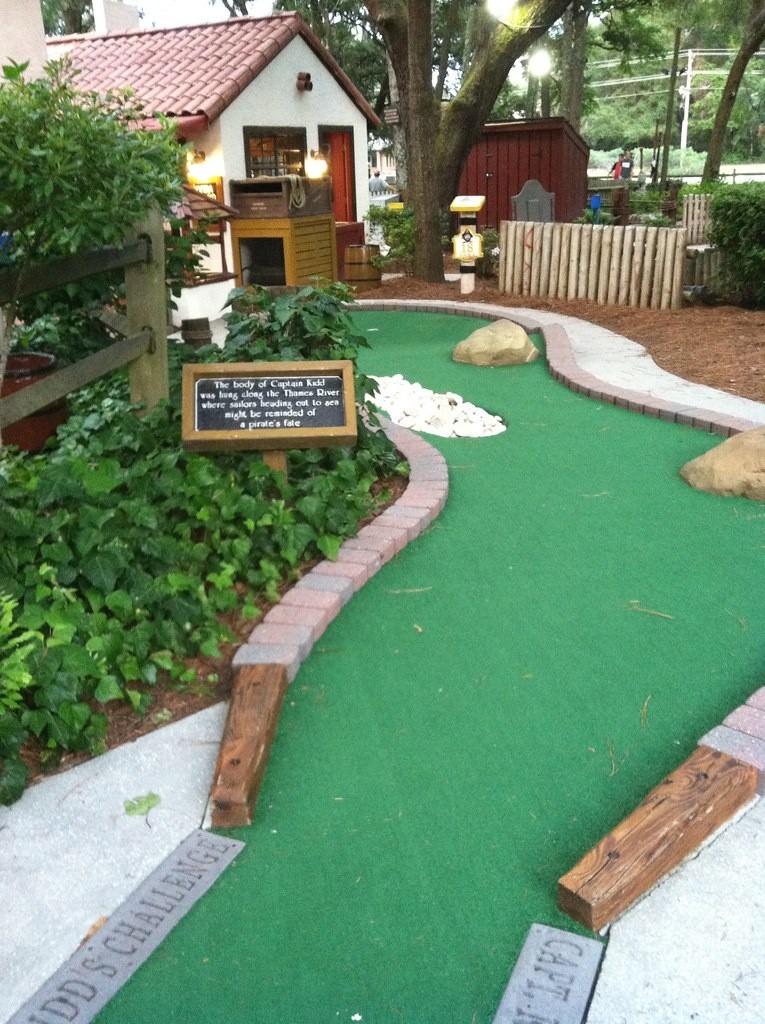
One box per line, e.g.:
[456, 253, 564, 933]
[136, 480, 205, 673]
[0, 352, 69, 452]
[344, 245, 381, 292]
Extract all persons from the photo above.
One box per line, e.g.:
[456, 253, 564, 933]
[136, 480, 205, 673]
[607, 151, 634, 179]
[368, 170, 397, 193]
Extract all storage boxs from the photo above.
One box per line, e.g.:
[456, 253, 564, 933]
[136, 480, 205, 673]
[230, 174, 333, 219]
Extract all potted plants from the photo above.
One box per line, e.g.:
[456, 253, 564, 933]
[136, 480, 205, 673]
[0, 54, 189, 460]
